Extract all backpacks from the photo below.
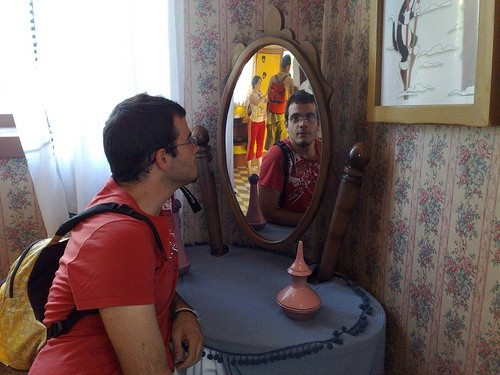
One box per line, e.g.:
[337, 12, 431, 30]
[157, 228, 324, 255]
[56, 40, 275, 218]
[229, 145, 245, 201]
[269, 75, 288, 114]
[0, 201, 168, 373]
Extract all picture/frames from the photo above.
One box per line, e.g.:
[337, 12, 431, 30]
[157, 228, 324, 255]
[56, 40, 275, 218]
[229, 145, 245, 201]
[368, 0, 500, 127]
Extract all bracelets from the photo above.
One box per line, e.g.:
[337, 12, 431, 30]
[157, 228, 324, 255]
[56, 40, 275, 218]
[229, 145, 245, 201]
[172, 307, 201, 322]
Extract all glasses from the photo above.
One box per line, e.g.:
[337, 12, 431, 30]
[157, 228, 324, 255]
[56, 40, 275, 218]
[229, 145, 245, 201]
[289, 114, 318, 123]
[164, 136, 197, 151]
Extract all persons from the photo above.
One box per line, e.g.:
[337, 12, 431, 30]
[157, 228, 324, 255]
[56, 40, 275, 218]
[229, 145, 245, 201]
[246, 54, 322, 226]
[27, 92, 204, 375]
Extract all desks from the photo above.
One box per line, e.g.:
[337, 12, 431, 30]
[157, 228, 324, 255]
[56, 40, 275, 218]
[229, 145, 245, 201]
[146, 242, 385, 375]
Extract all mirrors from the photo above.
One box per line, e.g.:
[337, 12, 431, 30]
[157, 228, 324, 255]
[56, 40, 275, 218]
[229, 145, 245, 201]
[192, 7, 365, 281]
